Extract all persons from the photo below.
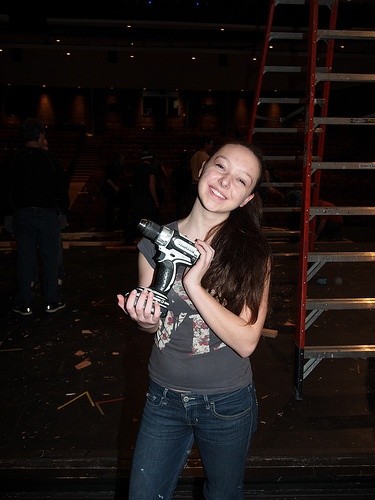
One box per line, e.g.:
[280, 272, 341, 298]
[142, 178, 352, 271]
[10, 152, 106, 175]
[6, 116, 67, 317]
[101, 132, 344, 244]
[115, 139, 271, 500]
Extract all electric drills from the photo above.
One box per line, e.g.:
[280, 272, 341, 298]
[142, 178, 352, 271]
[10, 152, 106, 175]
[124, 218, 201, 315]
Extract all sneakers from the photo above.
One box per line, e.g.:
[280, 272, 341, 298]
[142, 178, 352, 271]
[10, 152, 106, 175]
[11, 304, 32, 315]
[46, 302, 65, 312]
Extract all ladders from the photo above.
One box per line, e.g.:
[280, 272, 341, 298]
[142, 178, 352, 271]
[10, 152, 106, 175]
[247, 0, 375, 404]
